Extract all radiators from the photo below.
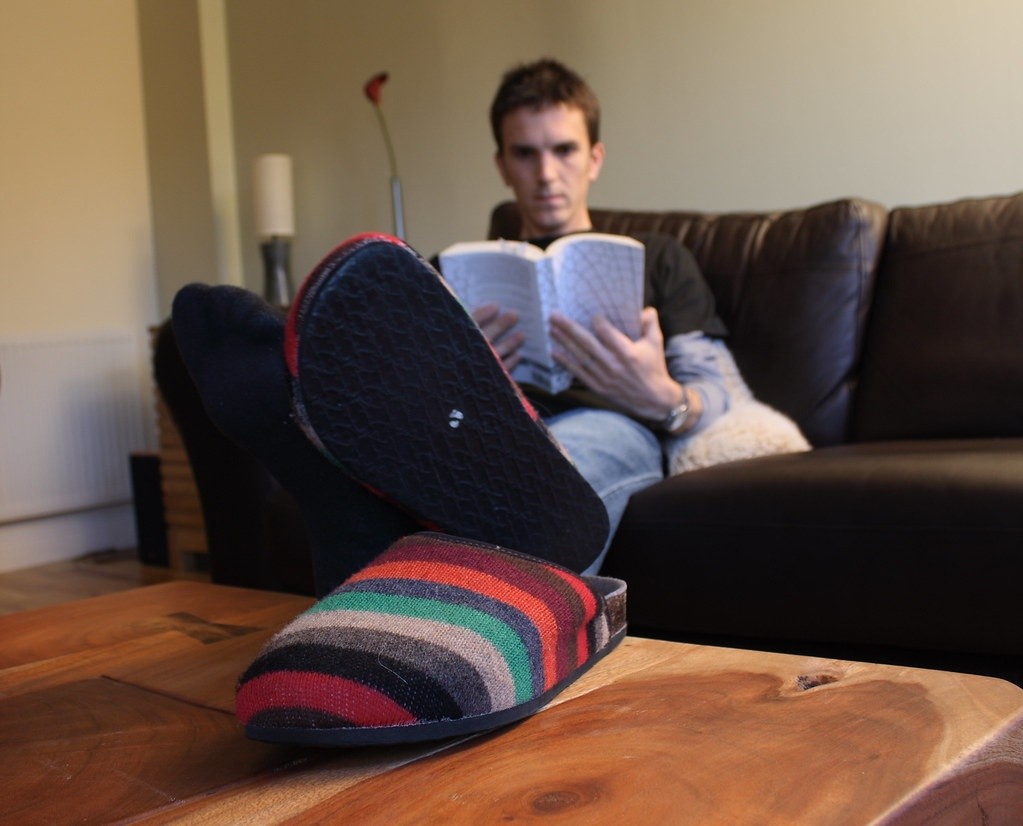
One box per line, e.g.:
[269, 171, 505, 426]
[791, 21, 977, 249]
[0, 332, 153, 523]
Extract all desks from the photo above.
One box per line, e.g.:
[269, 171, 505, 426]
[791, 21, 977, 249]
[0, 580, 1023, 826]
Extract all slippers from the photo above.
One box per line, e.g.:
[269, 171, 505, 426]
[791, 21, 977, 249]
[236, 531, 627, 746]
[284, 232, 611, 578]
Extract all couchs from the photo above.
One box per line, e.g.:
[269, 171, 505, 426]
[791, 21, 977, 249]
[148, 188, 1022, 688]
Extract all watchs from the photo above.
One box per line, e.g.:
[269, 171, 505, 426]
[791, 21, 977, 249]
[662, 387, 688, 432]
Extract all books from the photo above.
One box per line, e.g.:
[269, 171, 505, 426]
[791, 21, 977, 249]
[438, 233, 646, 395]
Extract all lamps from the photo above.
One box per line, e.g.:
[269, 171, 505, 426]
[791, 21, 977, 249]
[251, 152, 297, 311]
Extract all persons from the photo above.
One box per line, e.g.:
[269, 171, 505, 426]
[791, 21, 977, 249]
[153, 60, 727, 600]
